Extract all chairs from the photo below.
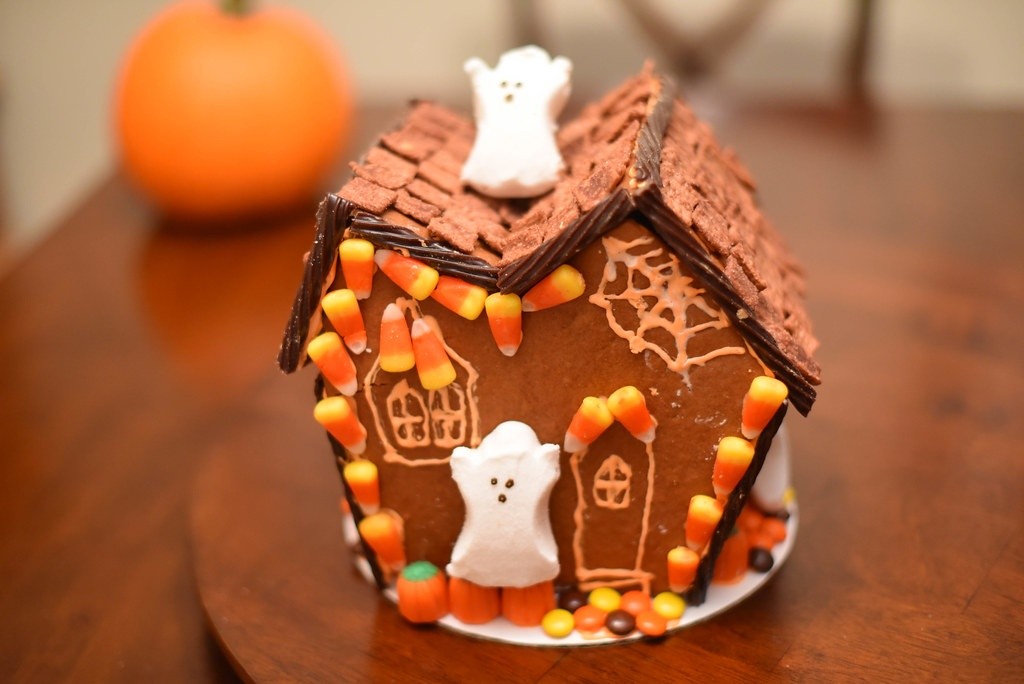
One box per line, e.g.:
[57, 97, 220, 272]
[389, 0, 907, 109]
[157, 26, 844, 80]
[507, 0, 875, 112]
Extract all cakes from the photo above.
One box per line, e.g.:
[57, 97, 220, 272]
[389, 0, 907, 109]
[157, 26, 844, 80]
[275, 43, 822, 638]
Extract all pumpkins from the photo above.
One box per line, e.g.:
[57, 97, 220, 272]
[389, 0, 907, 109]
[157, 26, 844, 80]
[113, 0, 356, 231]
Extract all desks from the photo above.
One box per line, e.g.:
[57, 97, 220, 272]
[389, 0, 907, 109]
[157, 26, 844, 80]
[0, 101, 1024, 684]
